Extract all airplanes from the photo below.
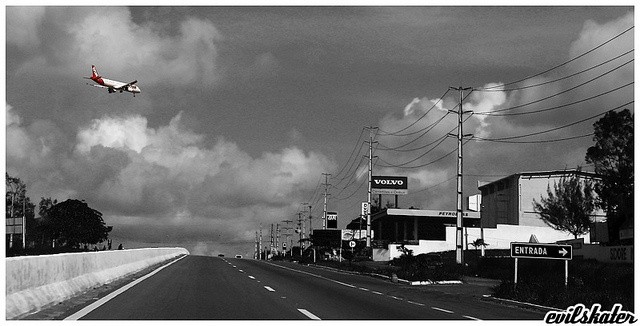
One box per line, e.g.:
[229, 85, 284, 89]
[82, 65, 141, 97]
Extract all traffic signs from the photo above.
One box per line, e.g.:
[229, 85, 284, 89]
[510, 242, 572, 260]
